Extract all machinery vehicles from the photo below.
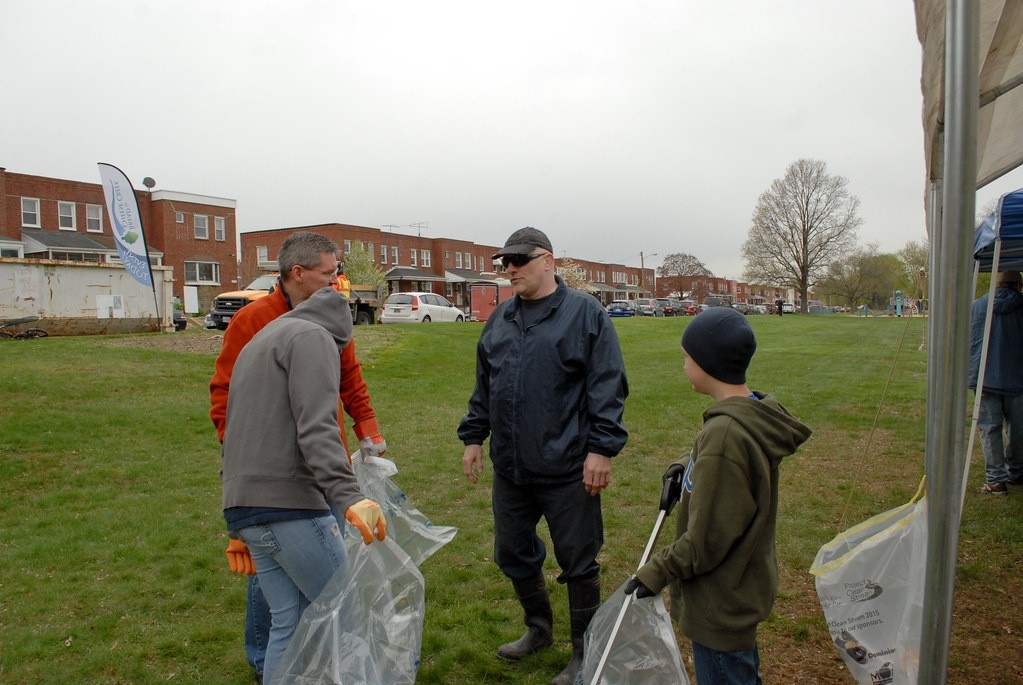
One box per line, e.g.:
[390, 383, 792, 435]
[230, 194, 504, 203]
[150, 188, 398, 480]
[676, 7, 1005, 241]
[210, 260, 382, 331]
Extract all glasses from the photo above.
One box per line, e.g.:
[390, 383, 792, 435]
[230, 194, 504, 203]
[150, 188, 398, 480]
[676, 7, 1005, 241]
[291, 264, 339, 281]
[502, 253, 547, 268]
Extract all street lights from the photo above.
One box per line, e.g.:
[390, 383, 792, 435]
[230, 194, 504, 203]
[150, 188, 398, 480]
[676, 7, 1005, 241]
[640, 251, 658, 298]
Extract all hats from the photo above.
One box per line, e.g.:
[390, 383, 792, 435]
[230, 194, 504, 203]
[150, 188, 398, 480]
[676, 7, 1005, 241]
[491, 226, 553, 260]
[681, 307, 756, 385]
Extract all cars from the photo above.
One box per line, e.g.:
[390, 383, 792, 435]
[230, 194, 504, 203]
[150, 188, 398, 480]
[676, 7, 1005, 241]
[377, 291, 465, 324]
[605, 297, 800, 317]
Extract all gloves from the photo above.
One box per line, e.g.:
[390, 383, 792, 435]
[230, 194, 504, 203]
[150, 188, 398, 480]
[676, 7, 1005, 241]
[662, 464, 684, 485]
[345, 499, 386, 545]
[352, 415, 386, 458]
[624, 577, 656, 599]
[226, 538, 257, 575]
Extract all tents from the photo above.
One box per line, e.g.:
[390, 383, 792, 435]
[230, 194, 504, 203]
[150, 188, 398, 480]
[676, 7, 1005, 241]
[960, 187, 1023, 520]
[917, 0, 1023, 685]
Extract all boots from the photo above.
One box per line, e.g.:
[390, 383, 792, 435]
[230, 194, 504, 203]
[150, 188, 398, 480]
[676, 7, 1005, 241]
[494, 587, 554, 663]
[550, 574, 600, 685]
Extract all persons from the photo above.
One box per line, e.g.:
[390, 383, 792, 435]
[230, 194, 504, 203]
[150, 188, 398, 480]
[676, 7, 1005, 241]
[209, 232, 388, 685]
[223, 287, 385, 685]
[624, 307, 812, 685]
[969, 270, 1023, 494]
[332, 269, 350, 301]
[457, 227, 630, 685]
[775, 296, 784, 316]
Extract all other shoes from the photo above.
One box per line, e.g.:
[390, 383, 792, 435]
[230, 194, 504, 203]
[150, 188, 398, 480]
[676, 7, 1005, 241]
[257, 671, 264, 685]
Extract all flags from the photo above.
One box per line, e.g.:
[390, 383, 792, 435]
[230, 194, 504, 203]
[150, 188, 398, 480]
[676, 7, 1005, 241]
[98, 163, 152, 287]
